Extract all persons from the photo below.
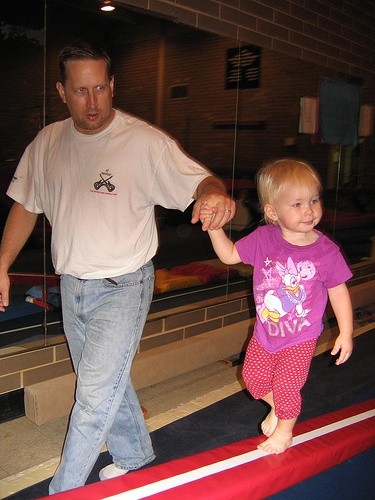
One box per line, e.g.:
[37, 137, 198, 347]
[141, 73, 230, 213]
[0, 42, 235, 495]
[199, 159, 354, 455]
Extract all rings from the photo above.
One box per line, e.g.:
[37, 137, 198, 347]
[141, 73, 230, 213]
[224, 210, 231, 214]
